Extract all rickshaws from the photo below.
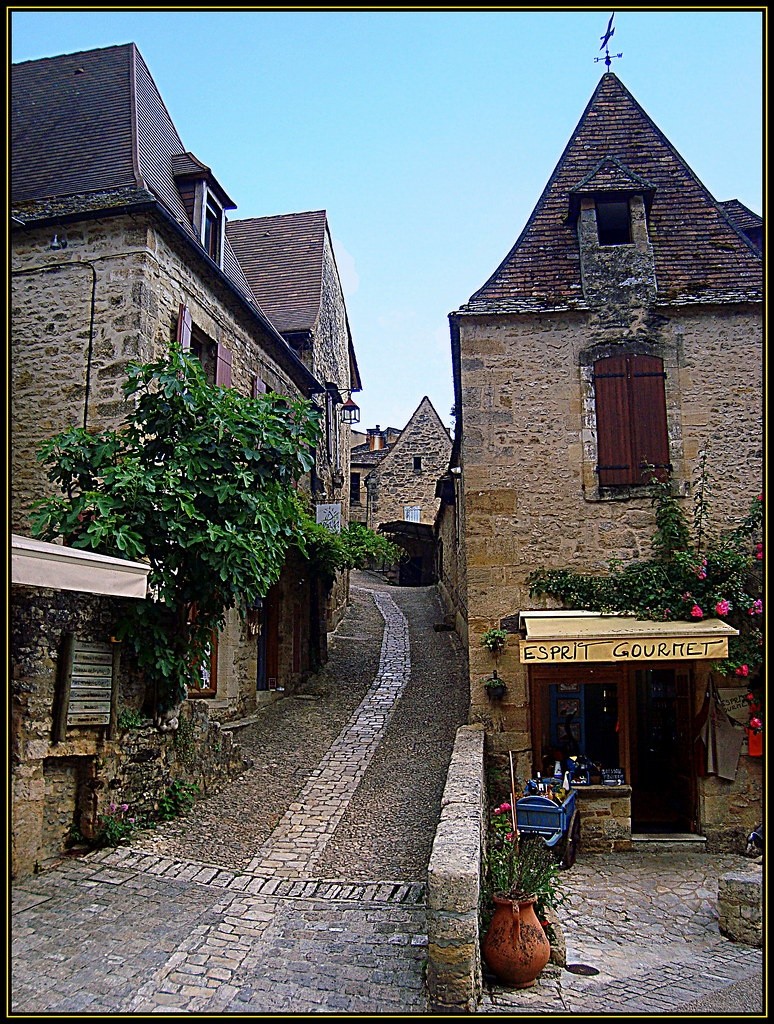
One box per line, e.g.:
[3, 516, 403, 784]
[515, 778, 581, 867]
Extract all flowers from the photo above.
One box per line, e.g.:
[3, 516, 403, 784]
[487, 801, 575, 907]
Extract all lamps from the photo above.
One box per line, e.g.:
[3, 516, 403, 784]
[307, 387, 362, 424]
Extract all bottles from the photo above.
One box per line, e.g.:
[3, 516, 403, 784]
[525, 771, 570, 806]
[601, 769, 625, 786]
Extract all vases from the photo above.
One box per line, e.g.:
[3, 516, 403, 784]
[486, 894, 552, 989]
